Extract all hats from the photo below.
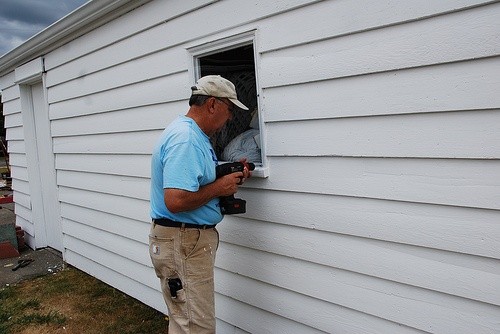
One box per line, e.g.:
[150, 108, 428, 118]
[191, 74, 250, 111]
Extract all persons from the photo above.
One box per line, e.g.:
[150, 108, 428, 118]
[148, 74, 253, 334]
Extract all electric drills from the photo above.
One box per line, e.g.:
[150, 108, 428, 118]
[215, 162, 262, 214]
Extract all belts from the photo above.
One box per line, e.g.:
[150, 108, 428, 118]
[152, 217, 217, 230]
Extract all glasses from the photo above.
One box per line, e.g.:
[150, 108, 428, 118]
[214, 97, 239, 113]
